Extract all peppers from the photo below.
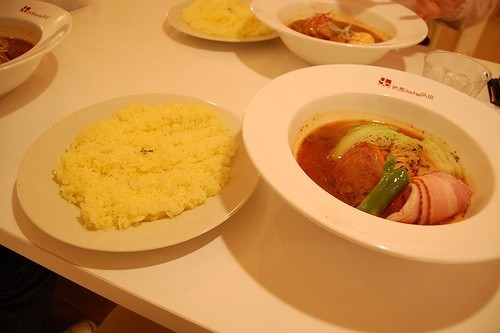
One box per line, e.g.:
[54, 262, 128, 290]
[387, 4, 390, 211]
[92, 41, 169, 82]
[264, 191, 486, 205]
[355, 156, 408, 215]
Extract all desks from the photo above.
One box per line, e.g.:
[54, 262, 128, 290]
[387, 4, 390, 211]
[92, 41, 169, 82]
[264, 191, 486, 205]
[0, 0, 500, 333]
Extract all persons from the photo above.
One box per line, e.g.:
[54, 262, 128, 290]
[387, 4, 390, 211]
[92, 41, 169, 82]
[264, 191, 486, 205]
[63, 320, 98, 333]
[407, 0, 500, 52]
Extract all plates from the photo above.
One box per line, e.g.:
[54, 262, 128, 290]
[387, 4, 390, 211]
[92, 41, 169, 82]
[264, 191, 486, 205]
[165, 0, 279, 42]
[16, 92, 261, 252]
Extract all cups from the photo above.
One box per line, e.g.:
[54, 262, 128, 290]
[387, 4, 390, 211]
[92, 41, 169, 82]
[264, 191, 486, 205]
[424, 49, 491, 98]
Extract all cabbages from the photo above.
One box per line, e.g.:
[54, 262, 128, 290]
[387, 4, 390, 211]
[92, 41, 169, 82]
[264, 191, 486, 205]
[326, 124, 462, 177]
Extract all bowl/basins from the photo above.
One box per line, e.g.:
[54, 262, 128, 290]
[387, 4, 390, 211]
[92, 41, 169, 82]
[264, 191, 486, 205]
[243, 64, 499, 265]
[0, 0, 74, 98]
[250, 0, 430, 64]
